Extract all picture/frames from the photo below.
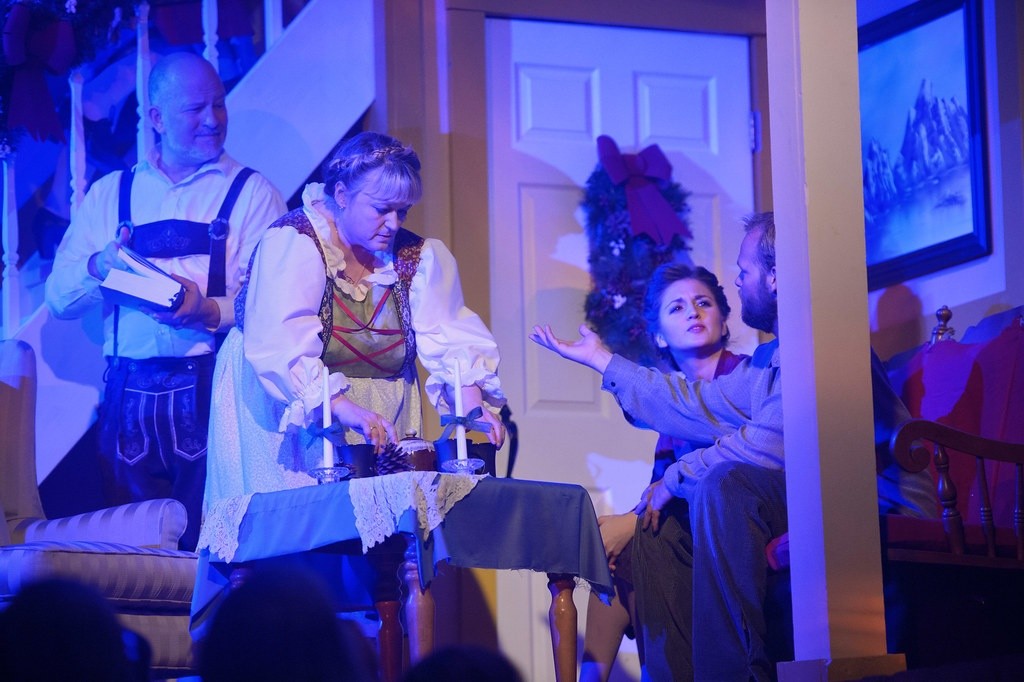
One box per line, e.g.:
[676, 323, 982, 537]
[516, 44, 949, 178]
[857, 1, 992, 289]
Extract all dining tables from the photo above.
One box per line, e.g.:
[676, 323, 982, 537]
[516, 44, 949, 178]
[188, 469, 619, 681]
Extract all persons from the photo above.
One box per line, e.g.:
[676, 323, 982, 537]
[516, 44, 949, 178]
[196, 569, 353, 682]
[529, 211, 937, 680]
[577, 260, 745, 682]
[44, 48, 288, 553]
[0, 569, 135, 682]
[189, 133, 510, 682]
[399, 641, 522, 682]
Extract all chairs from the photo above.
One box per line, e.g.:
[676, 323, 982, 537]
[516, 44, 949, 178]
[762, 312, 1023, 576]
[879, 337, 984, 503]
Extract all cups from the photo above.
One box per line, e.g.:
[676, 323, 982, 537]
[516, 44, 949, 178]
[433, 438, 472, 474]
[472, 441, 498, 479]
[338, 443, 375, 480]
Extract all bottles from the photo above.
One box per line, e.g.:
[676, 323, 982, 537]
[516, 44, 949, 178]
[396, 428, 437, 473]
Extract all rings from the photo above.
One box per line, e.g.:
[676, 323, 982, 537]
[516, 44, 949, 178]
[369, 426, 377, 430]
